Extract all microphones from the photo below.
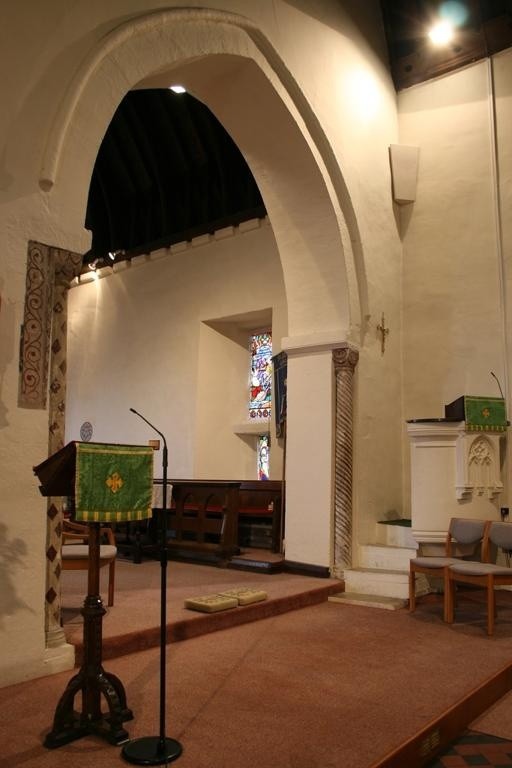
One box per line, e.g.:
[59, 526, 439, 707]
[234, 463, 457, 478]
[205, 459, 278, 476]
[128, 408, 167, 448]
[489, 372, 503, 398]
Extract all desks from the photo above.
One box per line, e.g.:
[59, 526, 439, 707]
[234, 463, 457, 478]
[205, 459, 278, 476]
[68, 482, 178, 564]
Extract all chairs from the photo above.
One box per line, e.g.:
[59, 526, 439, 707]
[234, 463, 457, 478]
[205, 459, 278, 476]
[448, 521, 512, 636]
[61, 518, 116, 608]
[408, 518, 490, 623]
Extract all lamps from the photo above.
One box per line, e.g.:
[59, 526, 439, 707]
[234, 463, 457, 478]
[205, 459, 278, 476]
[88, 256, 105, 271]
[108, 248, 129, 261]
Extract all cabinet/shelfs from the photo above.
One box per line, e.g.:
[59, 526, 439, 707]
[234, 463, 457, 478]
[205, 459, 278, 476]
[154, 478, 287, 574]
